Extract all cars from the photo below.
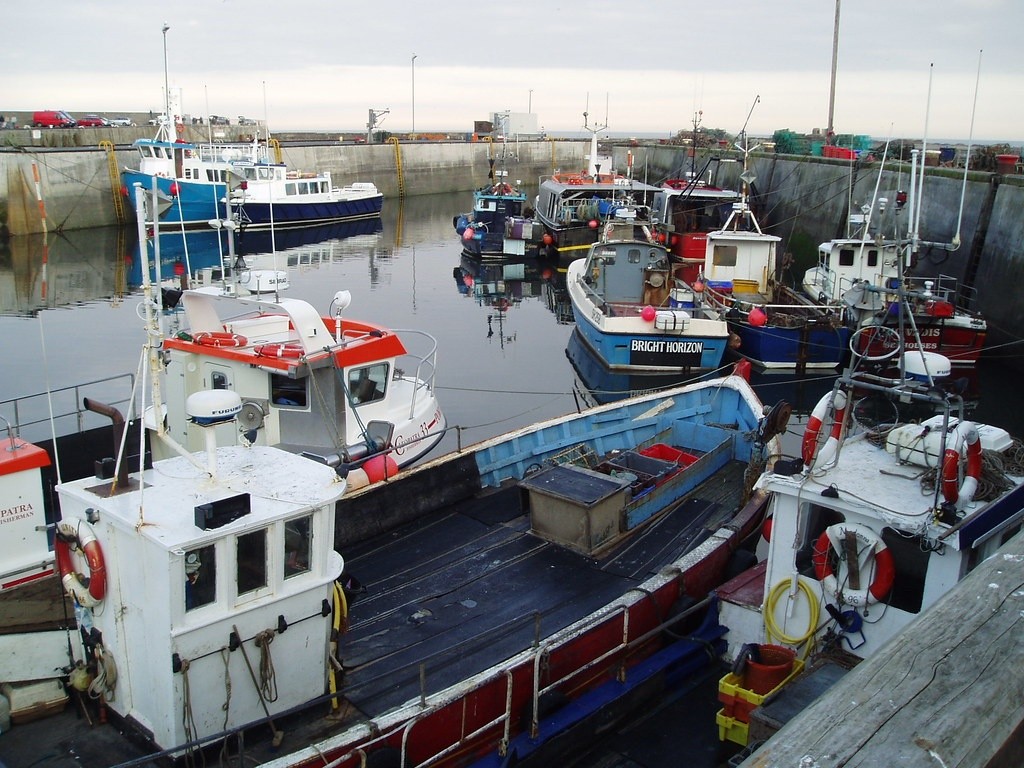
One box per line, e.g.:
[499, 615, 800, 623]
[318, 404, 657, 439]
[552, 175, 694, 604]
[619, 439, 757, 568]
[98, 117, 109, 127]
[109, 117, 132, 127]
[77, 116, 102, 126]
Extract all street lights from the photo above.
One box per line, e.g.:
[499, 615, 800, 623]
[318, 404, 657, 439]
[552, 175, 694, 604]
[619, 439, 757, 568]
[529, 89, 534, 114]
[162, 21, 171, 121]
[411, 55, 417, 138]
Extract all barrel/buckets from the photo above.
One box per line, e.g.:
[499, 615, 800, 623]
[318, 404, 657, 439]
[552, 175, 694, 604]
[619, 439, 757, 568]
[741, 644, 796, 694]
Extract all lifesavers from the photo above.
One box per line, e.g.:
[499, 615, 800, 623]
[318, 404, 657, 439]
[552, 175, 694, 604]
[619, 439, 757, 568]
[811, 521, 896, 608]
[192, 332, 249, 347]
[492, 182, 512, 196]
[254, 344, 305, 357]
[176, 123, 184, 133]
[943, 420, 984, 511]
[800, 388, 847, 470]
[54, 518, 108, 609]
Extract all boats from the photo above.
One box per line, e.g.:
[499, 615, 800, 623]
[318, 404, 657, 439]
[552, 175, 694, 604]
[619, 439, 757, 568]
[0, 46, 1024, 767]
[218, 165, 387, 236]
[224, 215, 387, 257]
[121, 234, 234, 292]
[118, 115, 269, 240]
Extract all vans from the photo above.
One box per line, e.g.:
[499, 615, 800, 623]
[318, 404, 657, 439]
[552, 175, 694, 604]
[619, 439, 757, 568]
[59, 111, 77, 128]
[33, 111, 73, 129]
